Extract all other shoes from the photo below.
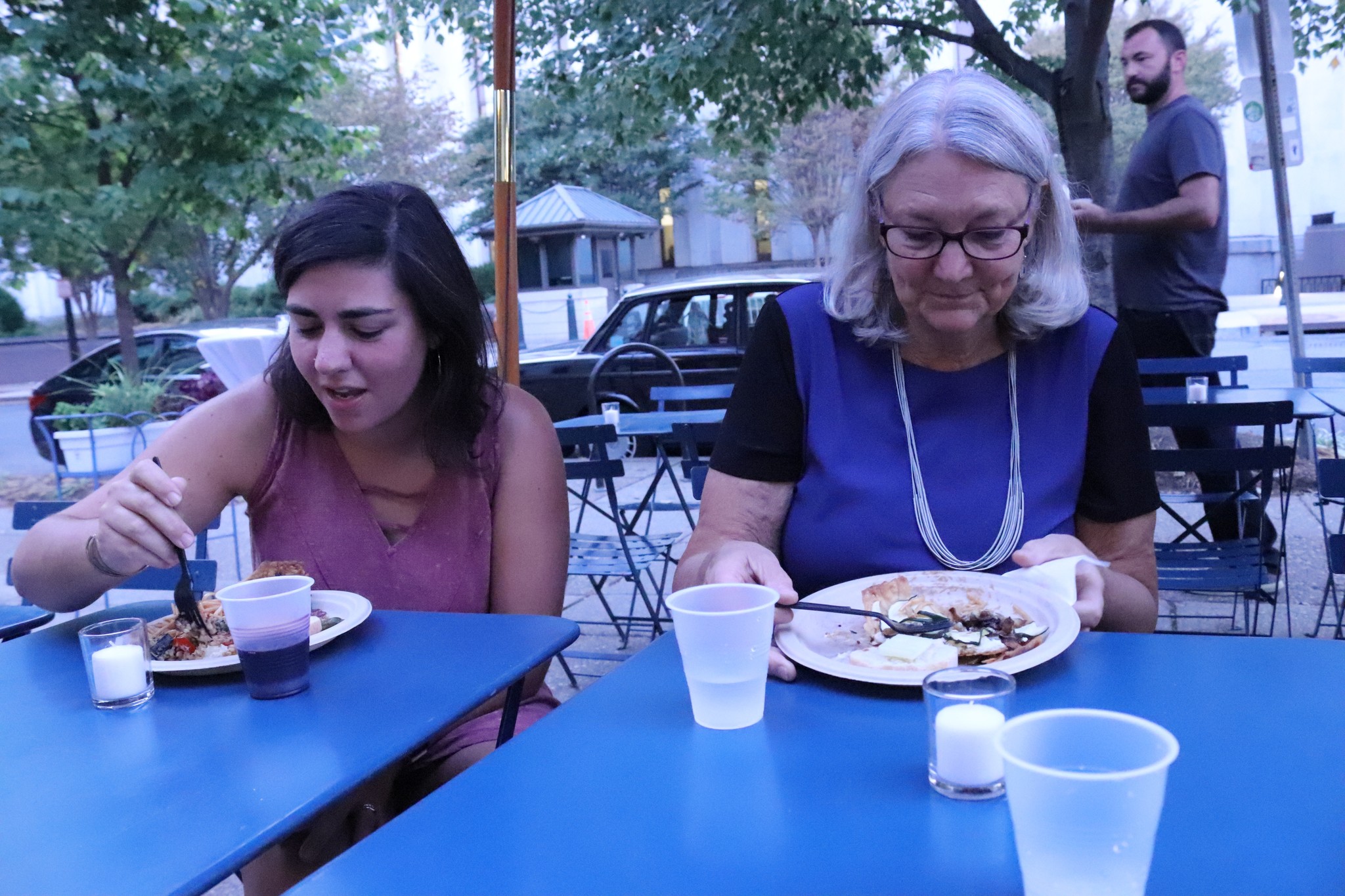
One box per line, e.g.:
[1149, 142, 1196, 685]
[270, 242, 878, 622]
[1260, 572, 1284, 591]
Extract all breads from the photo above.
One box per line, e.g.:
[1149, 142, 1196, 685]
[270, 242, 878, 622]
[309, 616, 322, 635]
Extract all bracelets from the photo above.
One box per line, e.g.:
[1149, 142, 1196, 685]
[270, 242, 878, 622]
[84, 534, 150, 577]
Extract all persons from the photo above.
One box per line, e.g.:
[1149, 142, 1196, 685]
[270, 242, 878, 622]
[1070, 19, 1284, 598]
[664, 65, 1164, 684]
[9, 182, 572, 895]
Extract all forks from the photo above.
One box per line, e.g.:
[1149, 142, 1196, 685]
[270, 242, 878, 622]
[151, 456, 212, 640]
[774, 600, 955, 633]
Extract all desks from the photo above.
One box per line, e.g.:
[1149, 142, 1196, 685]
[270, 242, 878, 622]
[1140, 387, 1334, 629]
[553, 414, 725, 646]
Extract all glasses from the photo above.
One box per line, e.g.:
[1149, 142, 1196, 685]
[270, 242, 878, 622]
[875, 184, 1035, 260]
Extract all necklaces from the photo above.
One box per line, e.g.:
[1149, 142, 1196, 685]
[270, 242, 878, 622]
[891, 338, 1026, 573]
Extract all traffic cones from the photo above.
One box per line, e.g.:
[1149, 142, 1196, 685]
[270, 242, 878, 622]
[580, 301, 594, 341]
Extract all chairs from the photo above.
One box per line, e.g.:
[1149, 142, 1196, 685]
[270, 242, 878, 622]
[8, 356, 1345, 687]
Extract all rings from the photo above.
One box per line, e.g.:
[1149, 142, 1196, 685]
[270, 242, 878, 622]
[355, 804, 376, 812]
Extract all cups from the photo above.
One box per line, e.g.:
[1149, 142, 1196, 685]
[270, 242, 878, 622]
[215, 575, 316, 701]
[1186, 376, 1209, 405]
[993, 707, 1181, 896]
[664, 583, 781, 730]
[601, 401, 620, 424]
[78, 617, 156, 711]
[921, 666, 1018, 801]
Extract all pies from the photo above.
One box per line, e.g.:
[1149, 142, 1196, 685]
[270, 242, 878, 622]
[849, 576, 1050, 672]
[237, 560, 310, 583]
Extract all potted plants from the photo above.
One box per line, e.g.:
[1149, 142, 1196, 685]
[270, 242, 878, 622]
[41, 340, 184, 468]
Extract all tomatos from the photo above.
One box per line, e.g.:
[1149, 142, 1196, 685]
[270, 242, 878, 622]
[223, 630, 234, 646]
[173, 636, 196, 654]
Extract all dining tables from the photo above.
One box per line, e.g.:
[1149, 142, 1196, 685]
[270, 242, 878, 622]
[0, 600, 579, 896]
[295, 574, 1345, 896]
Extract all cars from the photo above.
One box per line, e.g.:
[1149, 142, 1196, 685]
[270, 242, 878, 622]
[1, 316, 285, 475]
[477, 276, 827, 459]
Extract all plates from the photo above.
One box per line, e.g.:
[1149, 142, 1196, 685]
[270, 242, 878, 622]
[774, 569, 1081, 686]
[140, 589, 373, 678]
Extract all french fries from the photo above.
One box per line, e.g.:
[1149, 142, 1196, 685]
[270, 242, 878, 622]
[147, 591, 226, 640]
